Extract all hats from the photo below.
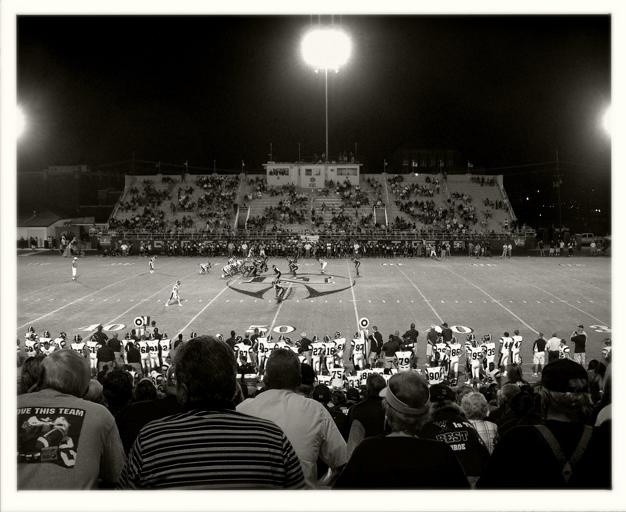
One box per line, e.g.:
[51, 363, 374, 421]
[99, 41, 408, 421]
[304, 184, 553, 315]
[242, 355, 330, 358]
[377, 370, 431, 416]
[535, 358, 589, 396]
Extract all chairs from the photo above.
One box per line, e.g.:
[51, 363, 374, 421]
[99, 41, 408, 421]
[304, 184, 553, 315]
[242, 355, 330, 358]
[107, 175, 516, 240]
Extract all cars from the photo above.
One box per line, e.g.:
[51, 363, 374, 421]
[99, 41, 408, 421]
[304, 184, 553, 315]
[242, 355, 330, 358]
[553, 222, 571, 232]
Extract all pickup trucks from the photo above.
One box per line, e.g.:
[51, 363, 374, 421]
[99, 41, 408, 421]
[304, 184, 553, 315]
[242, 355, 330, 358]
[577, 233, 604, 247]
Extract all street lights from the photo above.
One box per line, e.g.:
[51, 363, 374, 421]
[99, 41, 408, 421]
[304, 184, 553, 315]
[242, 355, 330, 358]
[298, 24, 353, 162]
